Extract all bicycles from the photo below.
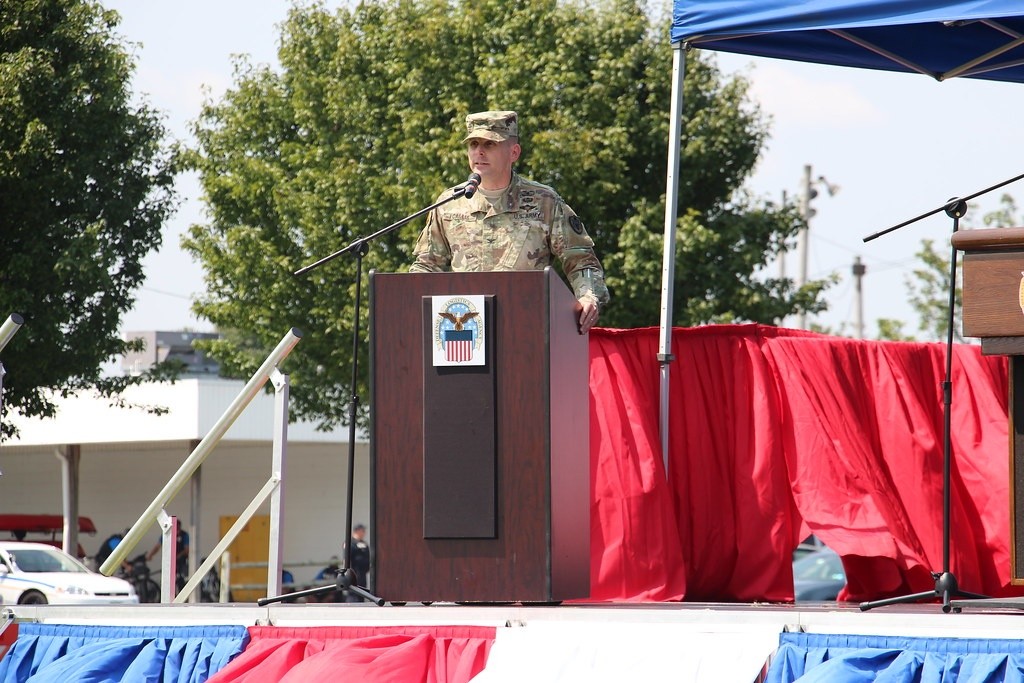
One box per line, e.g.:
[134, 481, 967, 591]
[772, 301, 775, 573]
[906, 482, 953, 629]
[122, 550, 162, 604]
[175, 558, 235, 604]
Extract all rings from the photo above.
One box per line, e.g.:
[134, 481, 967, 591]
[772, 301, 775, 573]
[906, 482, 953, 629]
[590, 319, 593, 322]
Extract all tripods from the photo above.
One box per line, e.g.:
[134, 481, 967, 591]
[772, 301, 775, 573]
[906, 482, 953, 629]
[257, 188, 468, 606]
[861, 173, 1024, 613]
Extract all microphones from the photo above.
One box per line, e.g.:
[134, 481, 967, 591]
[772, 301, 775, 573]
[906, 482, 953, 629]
[465, 173, 481, 199]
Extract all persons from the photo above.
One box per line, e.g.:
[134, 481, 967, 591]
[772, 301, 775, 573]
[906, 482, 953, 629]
[282, 524, 369, 603]
[409, 110, 609, 336]
[145, 520, 189, 604]
[95, 528, 132, 576]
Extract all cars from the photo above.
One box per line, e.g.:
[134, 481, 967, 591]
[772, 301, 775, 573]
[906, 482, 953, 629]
[0, 542, 140, 608]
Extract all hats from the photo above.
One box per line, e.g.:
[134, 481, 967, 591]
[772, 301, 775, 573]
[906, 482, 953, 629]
[461, 109, 519, 142]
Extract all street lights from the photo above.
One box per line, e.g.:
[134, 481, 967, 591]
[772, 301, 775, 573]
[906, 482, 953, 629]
[779, 196, 815, 330]
[802, 176, 838, 329]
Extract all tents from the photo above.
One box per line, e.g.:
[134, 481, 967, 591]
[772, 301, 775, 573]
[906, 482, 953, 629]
[656, 0, 1024, 481]
